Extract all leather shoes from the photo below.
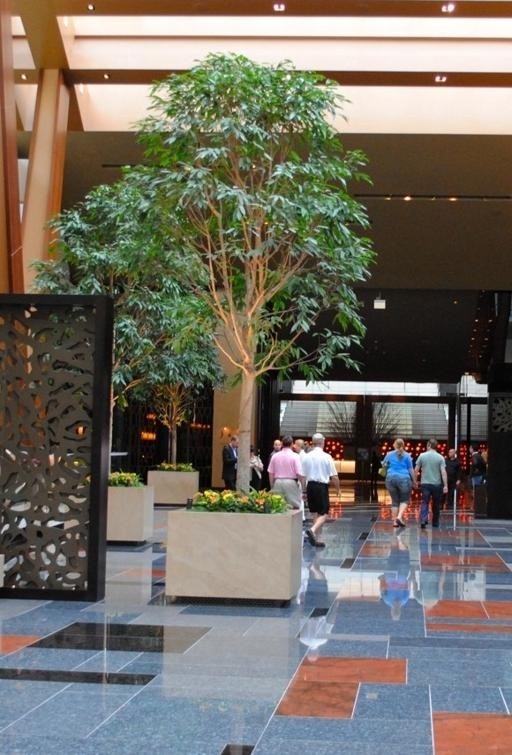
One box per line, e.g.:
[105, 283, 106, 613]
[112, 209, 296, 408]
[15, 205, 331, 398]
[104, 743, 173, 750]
[421, 522, 426, 528]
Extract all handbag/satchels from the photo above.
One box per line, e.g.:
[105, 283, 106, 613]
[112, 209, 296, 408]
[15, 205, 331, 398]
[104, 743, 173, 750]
[378, 468, 386, 477]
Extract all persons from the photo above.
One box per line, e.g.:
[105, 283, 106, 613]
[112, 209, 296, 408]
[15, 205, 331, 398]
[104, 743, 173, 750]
[221, 431, 488, 550]
[299, 529, 482, 663]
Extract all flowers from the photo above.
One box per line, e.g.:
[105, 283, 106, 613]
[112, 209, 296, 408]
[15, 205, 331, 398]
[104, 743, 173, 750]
[108, 468, 140, 487]
[156, 460, 195, 472]
[191, 490, 288, 512]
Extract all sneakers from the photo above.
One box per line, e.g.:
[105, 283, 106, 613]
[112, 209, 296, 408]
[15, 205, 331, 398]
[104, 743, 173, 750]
[303, 528, 325, 547]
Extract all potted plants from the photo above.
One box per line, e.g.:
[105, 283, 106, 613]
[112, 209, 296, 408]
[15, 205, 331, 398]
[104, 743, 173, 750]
[127, 295, 220, 506]
[113, 48, 379, 607]
[20, 183, 222, 547]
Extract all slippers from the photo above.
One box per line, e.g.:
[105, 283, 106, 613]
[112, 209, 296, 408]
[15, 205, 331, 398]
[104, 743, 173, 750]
[393, 519, 406, 527]
